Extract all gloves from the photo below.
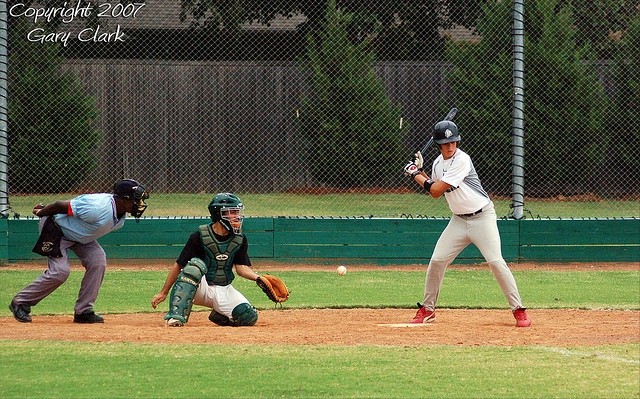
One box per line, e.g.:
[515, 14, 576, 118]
[403, 161, 420, 179]
[409, 151, 425, 172]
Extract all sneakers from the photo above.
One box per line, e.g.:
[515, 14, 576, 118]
[8, 300, 32, 323]
[513, 308, 531, 327]
[410, 302, 436, 324]
[73, 311, 104, 324]
[165, 318, 183, 328]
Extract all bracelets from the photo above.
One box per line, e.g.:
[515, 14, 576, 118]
[423, 179, 436, 191]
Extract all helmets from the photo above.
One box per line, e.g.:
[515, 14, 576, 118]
[432, 121, 461, 149]
[114, 178, 149, 220]
[209, 193, 244, 237]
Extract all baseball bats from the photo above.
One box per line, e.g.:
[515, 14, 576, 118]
[404, 108, 459, 177]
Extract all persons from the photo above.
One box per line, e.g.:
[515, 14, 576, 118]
[8, 180, 145, 324]
[150, 192, 266, 328]
[403, 121, 534, 328]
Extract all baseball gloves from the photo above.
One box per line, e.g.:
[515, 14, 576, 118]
[256, 275, 290, 308]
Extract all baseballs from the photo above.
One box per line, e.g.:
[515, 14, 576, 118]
[337, 266, 346, 276]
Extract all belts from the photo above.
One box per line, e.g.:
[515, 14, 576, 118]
[455, 209, 482, 217]
